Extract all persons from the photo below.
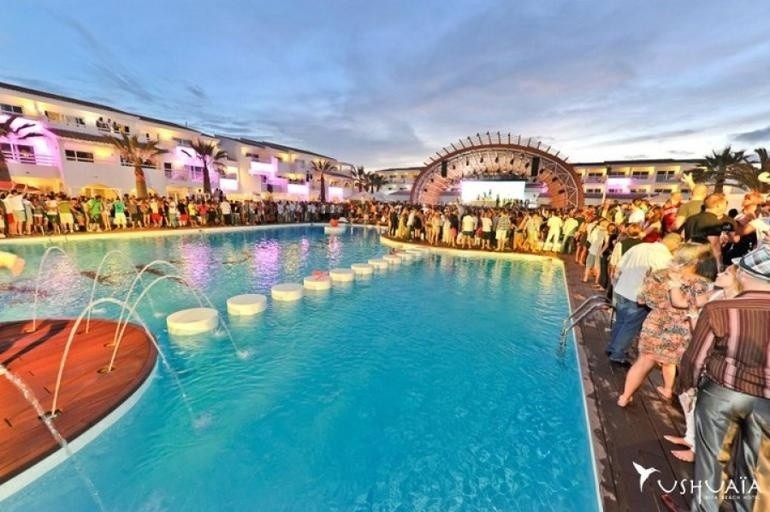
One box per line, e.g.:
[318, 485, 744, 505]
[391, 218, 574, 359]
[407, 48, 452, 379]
[0, 250, 26, 276]
[389, 189, 770, 253]
[575, 252, 770, 512]
[1, 179, 389, 239]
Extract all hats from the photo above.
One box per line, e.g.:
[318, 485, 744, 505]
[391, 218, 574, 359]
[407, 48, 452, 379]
[731, 244, 770, 281]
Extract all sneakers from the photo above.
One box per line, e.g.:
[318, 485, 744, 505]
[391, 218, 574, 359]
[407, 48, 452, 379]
[610, 357, 632, 367]
